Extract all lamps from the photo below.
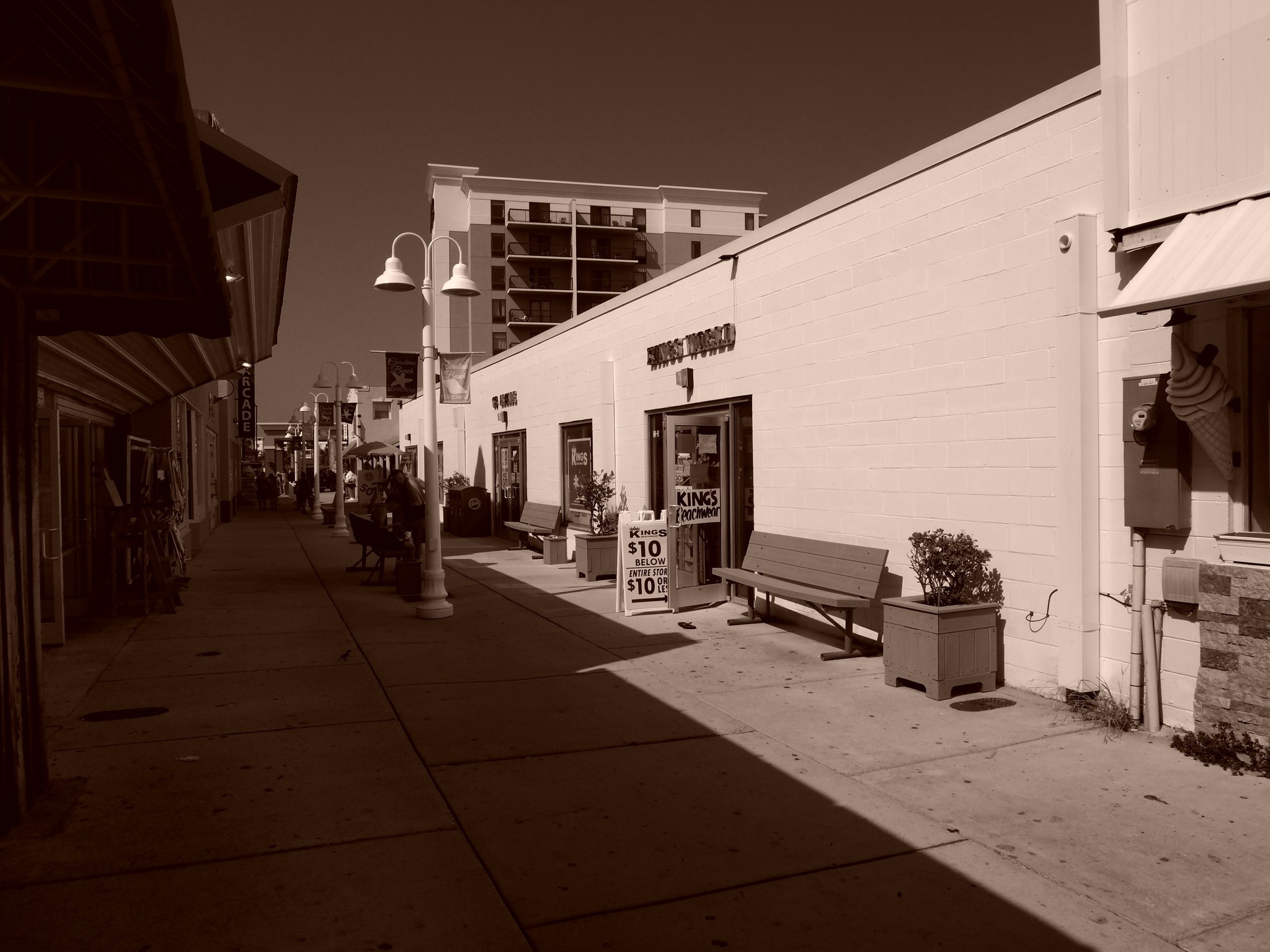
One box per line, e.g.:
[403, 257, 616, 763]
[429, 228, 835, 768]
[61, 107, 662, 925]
[235, 417, 239, 425]
[223, 259, 244, 284]
[497, 410, 508, 423]
[405, 434, 411, 441]
[676, 367, 694, 390]
[213, 380, 234, 404]
[718, 254, 738, 262]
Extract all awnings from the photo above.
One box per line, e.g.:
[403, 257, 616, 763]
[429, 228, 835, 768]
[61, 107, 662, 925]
[0, 1, 233, 336]
[342, 441, 357, 459]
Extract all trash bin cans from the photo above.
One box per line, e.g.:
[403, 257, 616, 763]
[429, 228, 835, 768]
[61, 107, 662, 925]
[448, 485, 493, 538]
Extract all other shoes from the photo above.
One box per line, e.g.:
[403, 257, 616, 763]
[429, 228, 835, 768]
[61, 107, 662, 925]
[262, 507, 268, 511]
[274, 506, 278, 510]
[259, 507, 262, 510]
[270, 506, 273, 510]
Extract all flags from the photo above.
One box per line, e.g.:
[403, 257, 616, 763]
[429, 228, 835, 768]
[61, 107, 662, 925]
[302, 423, 314, 440]
[292, 436, 302, 450]
[341, 402, 358, 424]
[385, 351, 419, 400]
[318, 402, 335, 426]
[306, 429, 314, 449]
[438, 351, 472, 404]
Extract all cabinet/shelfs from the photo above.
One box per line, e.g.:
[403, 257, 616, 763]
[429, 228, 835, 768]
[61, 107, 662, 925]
[107, 504, 191, 615]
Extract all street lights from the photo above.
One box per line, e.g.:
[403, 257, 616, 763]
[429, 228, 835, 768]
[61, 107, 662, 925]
[297, 393, 330, 521]
[371, 230, 483, 622]
[283, 408, 314, 499]
[312, 361, 363, 538]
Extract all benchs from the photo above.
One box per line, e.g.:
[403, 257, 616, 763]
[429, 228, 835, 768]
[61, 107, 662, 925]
[712, 532, 888, 661]
[503, 500, 562, 559]
[319, 493, 346, 529]
[350, 511, 420, 588]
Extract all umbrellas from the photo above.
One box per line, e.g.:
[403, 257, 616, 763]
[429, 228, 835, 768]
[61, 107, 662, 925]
[347, 441, 406, 497]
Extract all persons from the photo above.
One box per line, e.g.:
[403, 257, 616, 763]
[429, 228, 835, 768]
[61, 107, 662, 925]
[265, 473, 284, 499]
[266, 474, 278, 510]
[370, 493, 415, 573]
[1096, 195, 1270, 319]
[256, 471, 269, 510]
[294, 471, 313, 514]
[383, 468, 426, 564]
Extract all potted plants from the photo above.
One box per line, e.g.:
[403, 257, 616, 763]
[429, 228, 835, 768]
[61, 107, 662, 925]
[879, 529, 1004, 702]
[573, 469, 620, 581]
[439, 470, 471, 532]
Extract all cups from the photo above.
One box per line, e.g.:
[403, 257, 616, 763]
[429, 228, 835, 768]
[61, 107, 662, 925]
[404, 531, 411, 540]
